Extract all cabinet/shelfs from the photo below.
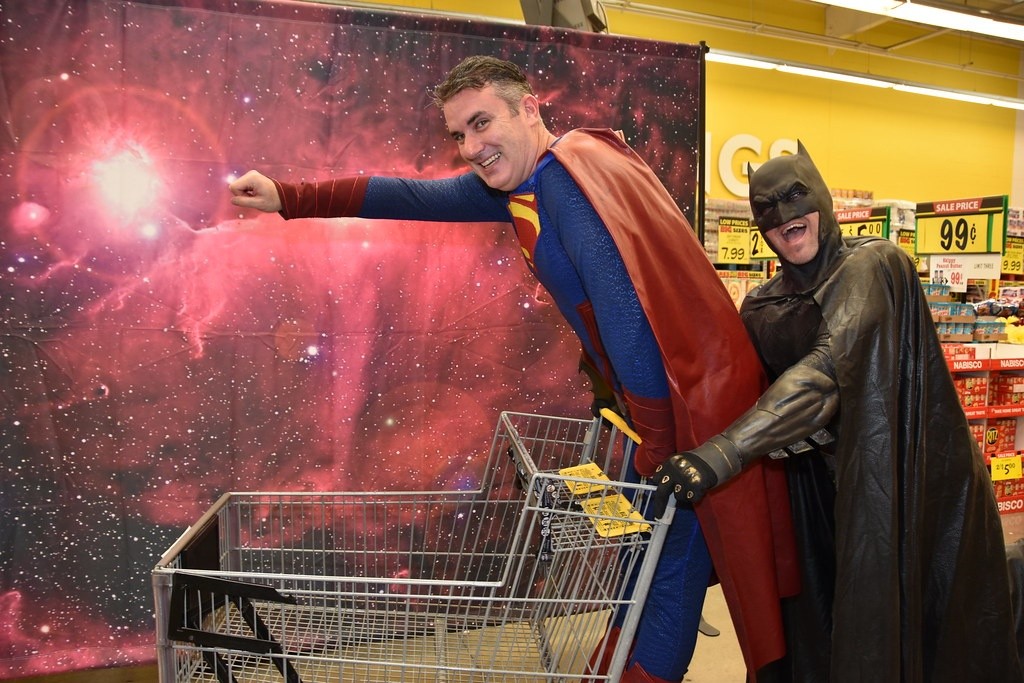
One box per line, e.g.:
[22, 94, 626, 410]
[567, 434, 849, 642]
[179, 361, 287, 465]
[964, 279, 1024, 318]
[920, 343, 1024, 515]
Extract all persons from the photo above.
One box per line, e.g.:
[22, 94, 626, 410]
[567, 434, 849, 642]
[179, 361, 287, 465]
[230, 56, 764, 683]
[650, 137, 1023, 683]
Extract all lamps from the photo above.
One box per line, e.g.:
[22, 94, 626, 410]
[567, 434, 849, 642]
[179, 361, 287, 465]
[705, 49, 1024, 111]
[811, 0, 1024, 43]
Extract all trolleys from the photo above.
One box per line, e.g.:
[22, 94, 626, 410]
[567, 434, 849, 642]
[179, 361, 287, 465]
[153, 408, 676, 683]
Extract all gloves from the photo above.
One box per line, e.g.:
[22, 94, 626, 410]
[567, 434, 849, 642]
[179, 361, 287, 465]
[653, 453, 718, 519]
